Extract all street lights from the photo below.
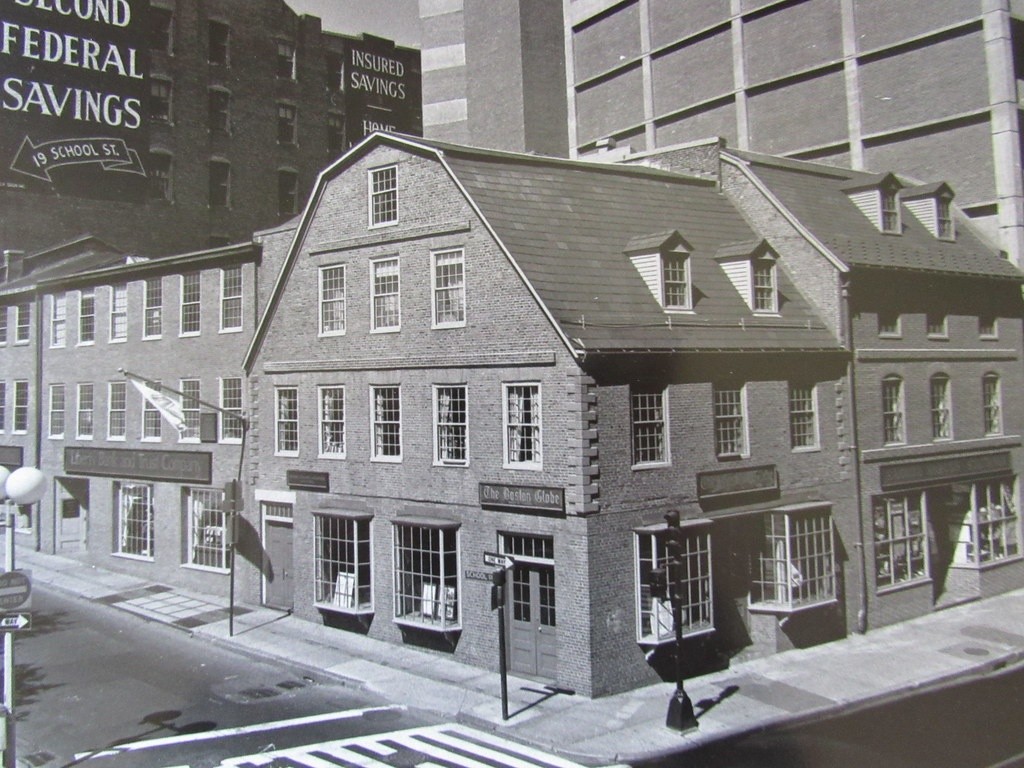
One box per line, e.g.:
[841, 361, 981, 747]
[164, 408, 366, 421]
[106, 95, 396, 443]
[0, 464, 48, 766]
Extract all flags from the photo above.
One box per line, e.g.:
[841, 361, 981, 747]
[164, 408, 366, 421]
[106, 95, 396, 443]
[129, 377, 188, 433]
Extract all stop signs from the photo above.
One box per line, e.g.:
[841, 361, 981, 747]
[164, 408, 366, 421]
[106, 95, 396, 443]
[0, 571, 34, 613]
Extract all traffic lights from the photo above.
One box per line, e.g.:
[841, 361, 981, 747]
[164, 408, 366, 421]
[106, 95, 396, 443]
[663, 510, 681, 555]
